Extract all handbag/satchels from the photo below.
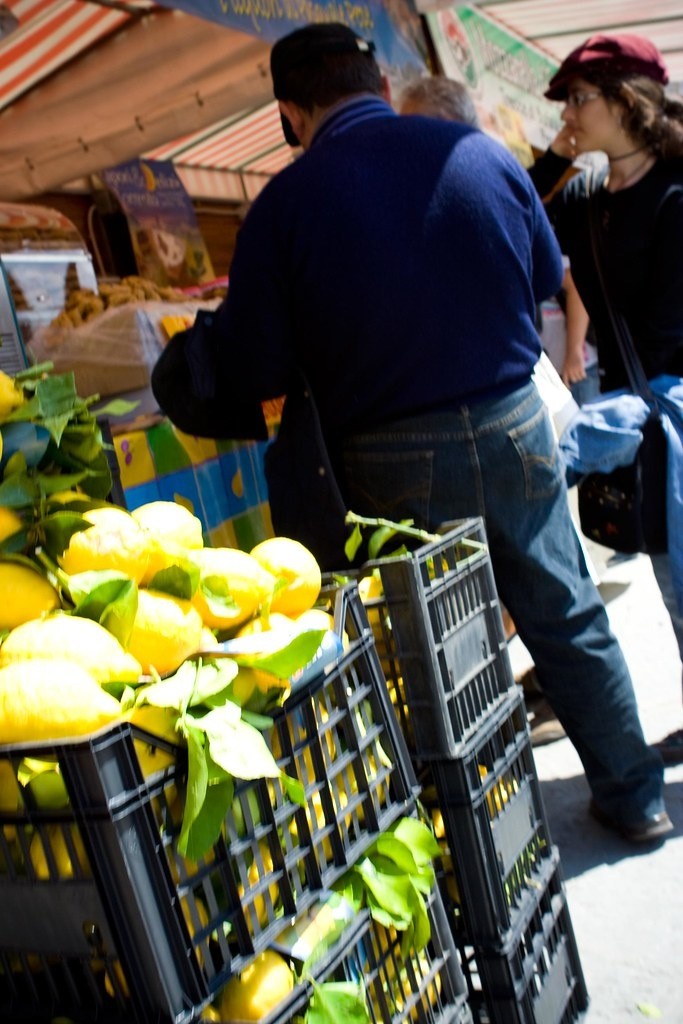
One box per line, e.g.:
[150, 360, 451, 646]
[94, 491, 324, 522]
[577, 412, 667, 556]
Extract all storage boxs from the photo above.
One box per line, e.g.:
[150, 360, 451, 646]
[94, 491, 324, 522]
[1, 384, 589, 1024]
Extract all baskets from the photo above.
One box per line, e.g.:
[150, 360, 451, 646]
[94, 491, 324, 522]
[0, 515, 589, 1024]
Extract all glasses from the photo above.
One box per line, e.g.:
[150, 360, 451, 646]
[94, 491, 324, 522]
[565, 89, 603, 108]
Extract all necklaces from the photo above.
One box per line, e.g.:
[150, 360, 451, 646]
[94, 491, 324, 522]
[607, 143, 648, 161]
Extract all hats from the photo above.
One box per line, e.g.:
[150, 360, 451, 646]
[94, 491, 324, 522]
[270, 22, 376, 147]
[543, 34, 669, 102]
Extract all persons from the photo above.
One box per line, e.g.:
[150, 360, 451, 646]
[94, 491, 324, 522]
[216, 20, 676, 848]
[401, 75, 683, 767]
[516, 27, 682, 769]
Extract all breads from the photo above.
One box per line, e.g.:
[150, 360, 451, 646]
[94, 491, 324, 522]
[41, 275, 229, 350]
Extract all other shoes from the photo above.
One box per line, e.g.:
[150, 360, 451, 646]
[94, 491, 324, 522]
[588, 794, 675, 841]
[526, 699, 567, 745]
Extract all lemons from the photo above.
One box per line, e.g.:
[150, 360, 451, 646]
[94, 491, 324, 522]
[0, 369, 524, 1024]
